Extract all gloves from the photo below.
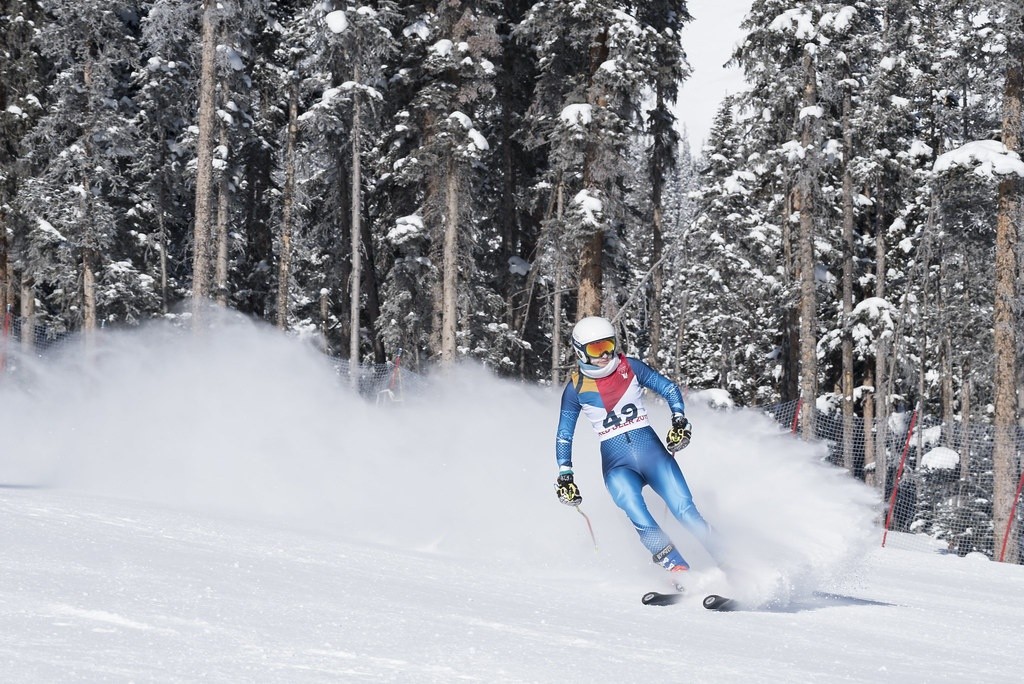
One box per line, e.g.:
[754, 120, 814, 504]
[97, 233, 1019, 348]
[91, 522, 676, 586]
[554, 474, 582, 507]
[666, 417, 692, 452]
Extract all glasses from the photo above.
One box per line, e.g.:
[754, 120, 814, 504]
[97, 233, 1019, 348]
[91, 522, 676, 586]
[585, 336, 617, 358]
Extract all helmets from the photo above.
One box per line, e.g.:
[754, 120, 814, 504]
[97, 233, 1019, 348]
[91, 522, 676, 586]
[571, 316, 621, 378]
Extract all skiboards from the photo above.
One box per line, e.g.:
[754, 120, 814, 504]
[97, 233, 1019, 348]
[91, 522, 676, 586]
[642, 591, 738, 612]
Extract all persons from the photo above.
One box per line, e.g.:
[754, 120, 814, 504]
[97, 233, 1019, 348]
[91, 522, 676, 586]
[554, 317, 755, 590]
